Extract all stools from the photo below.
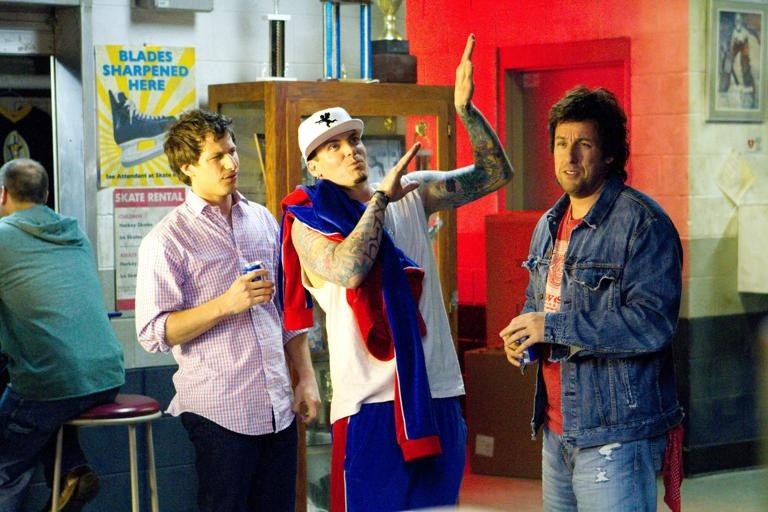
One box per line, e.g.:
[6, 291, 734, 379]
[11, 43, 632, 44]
[51, 393, 164, 512]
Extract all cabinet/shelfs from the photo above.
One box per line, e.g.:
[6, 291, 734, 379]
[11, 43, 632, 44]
[206, 81, 459, 512]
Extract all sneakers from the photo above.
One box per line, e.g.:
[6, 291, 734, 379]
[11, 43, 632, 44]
[58, 464, 100, 510]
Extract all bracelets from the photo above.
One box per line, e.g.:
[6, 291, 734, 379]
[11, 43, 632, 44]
[373, 190, 391, 204]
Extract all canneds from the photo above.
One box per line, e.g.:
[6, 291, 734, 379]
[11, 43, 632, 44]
[511, 325, 540, 366]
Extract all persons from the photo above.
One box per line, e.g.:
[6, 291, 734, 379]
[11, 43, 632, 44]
[135, 108, 322, 512]
[725, 12, 761, 108]
[0, 158, 127, 511]
[290, 33, 515, 511]
[498, 86, 685, 511]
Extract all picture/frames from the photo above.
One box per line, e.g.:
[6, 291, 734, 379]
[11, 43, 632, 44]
[703, 0, 768, 122]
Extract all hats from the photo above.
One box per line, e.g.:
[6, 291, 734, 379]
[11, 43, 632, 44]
[298, 107, 365, 163]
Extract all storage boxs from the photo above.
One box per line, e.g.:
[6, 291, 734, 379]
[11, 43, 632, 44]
[465, 344, 541, 480]
